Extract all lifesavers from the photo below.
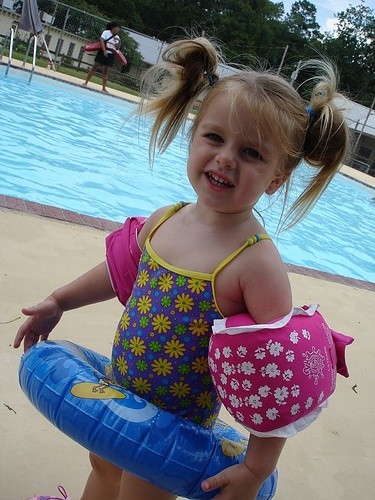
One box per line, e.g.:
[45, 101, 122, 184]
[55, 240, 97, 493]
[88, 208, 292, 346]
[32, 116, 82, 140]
[208, 302, 355, 439]
[105, 216, 148, 307]
[82, 42, 127, 65]
[17, 339, 279, 500]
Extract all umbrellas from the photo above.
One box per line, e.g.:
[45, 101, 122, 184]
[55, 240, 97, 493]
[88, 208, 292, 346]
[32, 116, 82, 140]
[19, 0, 57, 71]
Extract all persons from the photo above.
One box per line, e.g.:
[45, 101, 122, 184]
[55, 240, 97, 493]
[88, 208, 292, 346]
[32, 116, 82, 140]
[83, 22, 123, 93]
[14, 38, 354, 499]
